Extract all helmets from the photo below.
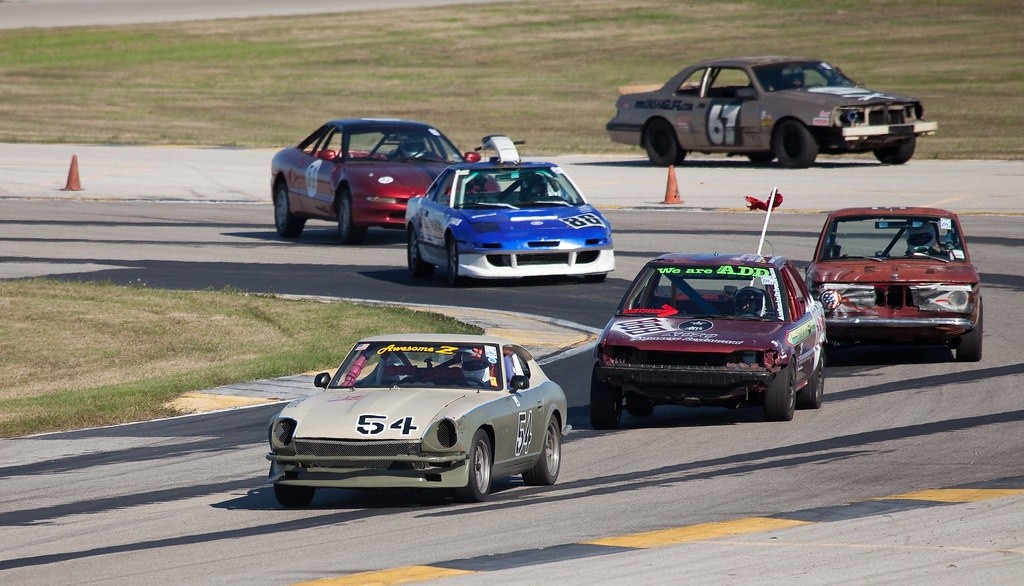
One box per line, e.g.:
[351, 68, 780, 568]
[733, 286, 766, 318]
[782, 67, 805, 90]
[461, 350, 490, 384]
[520, 174, 547, 202]
[399, 135, 427, 159]
[907, 220, 937, 255]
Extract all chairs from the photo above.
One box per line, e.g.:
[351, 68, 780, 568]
[759, 289, 775, 316]
[535, 175, 548, 196]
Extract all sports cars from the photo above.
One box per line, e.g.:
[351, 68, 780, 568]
[806, 208, 985, 364]
[264, 333, 571, 512]
[590, 254, 828, 429]
[404, 135, 615, 289]
[606, 56, 938, 169]
[269, 119, 502, 247]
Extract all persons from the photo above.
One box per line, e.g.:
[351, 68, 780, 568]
[454, 354, 498, 388]
[904, 221, 941, 256]
[388, 133, 442, 160]
[782, 67, 804, 88]
[732, 286, 765, 317]
[521, 174, 548, 202]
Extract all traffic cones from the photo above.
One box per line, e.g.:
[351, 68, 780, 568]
[661, 166, 686, 204]
[61, 154, 86, 191]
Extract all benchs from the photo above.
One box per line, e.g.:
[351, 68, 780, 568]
[386, 366, 461, 381]
